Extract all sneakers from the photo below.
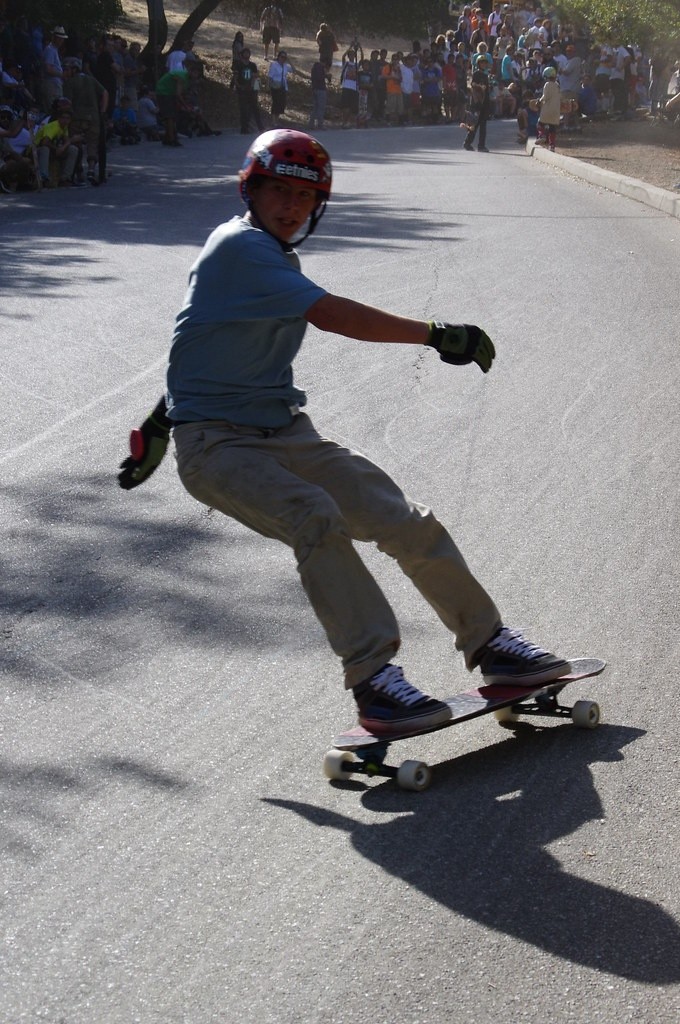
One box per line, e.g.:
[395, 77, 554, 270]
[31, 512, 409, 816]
[480, 628, 572, 687]
[353, 664, 453, 731]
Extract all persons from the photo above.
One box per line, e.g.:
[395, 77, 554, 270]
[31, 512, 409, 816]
[115, 130, 570, 734]
[0, 0, 680, 195]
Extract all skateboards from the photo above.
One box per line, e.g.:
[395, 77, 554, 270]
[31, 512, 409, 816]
[322, 656, 607, 792]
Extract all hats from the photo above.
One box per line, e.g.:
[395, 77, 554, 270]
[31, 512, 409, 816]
[565, 44, 576, 52]
[50, 26, 69, 38]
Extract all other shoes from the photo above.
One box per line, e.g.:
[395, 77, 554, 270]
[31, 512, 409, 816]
[610, 113, 627, 123]
[550, 145, 555, 152]
[78, 179, 87, 186]
[463, 144, 475, 151]
[535, 137, 547, 144]
[86, 171, 98, 185]
[478, 146, 489, 152]
[517, 130, 528, 139]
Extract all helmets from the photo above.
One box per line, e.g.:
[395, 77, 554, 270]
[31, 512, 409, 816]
[542, 66, 557, 78]
[238, 129, 332, 210]
[476, 55, 488, 66]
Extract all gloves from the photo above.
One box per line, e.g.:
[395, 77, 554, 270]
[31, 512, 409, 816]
[425, 321, 495, 374]
[117, 396, 173, 490]
